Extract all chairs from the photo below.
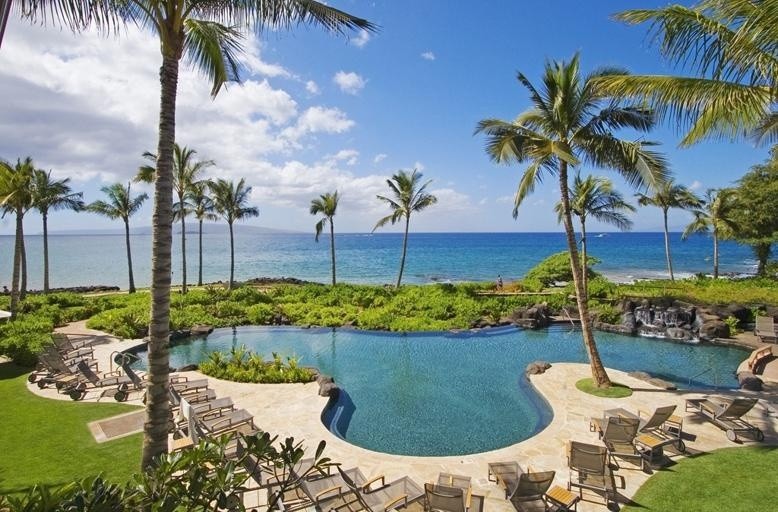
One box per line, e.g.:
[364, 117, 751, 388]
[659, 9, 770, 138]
[755, 315, 778, 344]
[165, 377, 778, 512]
[28, 333, 149, 403]
